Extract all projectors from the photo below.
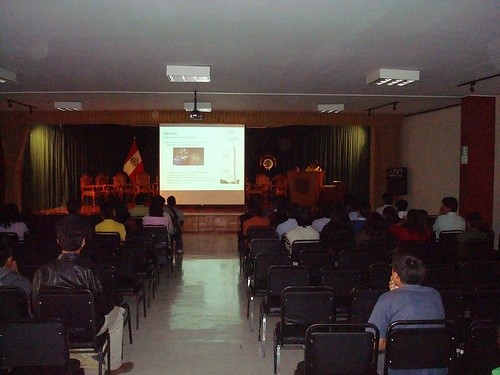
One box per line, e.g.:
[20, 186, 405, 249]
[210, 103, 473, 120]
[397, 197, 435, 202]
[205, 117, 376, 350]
[190, 113, 205, 121]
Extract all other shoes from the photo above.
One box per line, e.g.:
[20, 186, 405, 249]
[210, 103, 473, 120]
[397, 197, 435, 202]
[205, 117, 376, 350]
[178, 249, 184, 254]
[105, 362, 134, 375]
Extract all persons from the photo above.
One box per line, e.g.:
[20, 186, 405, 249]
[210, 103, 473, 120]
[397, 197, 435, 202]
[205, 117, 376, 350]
[0, 243, 41, 319]
[0, 192, 185, 247]
[366, 255, 448, 375]
[304, 160, 322, 172]
[32, 214, 134, 375]
[239, 192, 494, 262]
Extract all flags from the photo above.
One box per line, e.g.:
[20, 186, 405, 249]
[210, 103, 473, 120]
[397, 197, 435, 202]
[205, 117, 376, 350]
[123, 139, 146, 188]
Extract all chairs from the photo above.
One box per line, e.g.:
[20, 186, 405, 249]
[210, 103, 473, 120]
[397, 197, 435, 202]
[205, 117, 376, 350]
[0, 208, 185, 375]
[244, 173, 287, 200]
[80, 173, 159, 206]
[238, 219, 500, 375]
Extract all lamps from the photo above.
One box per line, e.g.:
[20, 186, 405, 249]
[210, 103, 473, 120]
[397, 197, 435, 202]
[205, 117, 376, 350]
[365, 68, 419, 86]
[29, 106, 33, 113]
[393, 102, 397, 110]
[368, 110, 371, 116]
[7, 100, 12, 108]
[0, 67, 16, 85]
[469, 81, 475, 92]
[183, 103, 212, 113]
[166, 65, 210, 83]
[53, 101, 86, 112]
[313, 104, 344, 113]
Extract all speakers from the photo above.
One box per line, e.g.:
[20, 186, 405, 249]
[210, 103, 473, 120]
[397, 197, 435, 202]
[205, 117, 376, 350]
[387, 167, 407, 194]
